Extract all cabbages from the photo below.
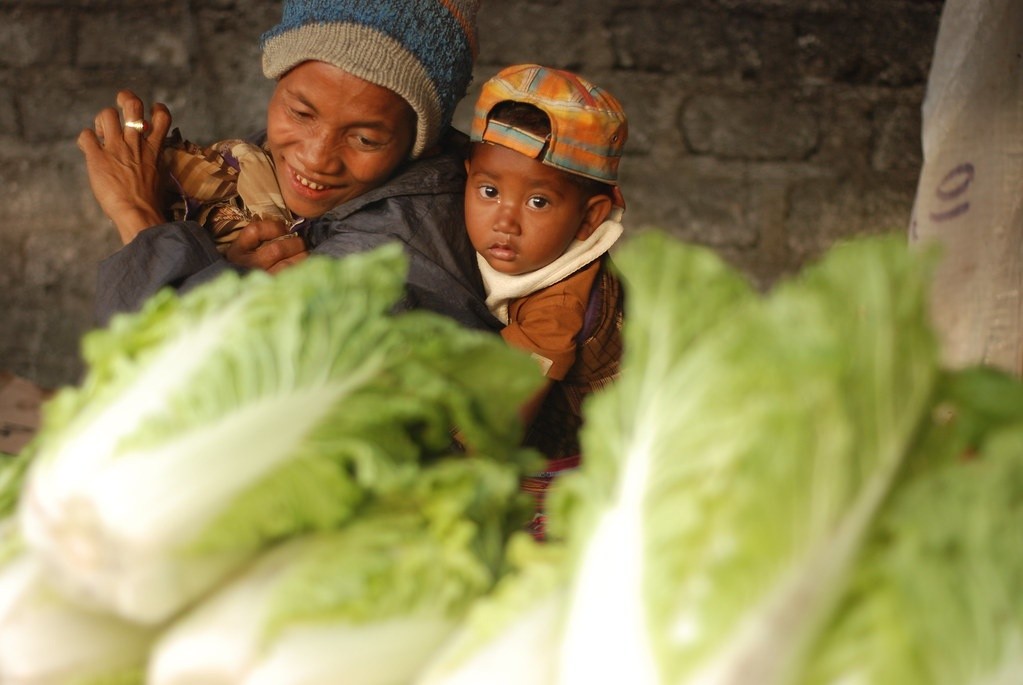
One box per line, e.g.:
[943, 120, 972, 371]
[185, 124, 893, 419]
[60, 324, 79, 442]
[0, 230, 1023, 685]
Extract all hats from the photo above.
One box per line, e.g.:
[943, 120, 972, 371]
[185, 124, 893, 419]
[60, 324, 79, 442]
[262, 0, 483, 161]
[470, 63, 628, 185]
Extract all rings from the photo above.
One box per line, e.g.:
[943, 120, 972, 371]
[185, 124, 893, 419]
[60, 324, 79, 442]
[125, 119, 148, 133]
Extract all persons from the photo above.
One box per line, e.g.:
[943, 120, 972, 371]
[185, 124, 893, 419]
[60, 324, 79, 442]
[451, 62, 622, 539]
[78, 0, 502, 337]
